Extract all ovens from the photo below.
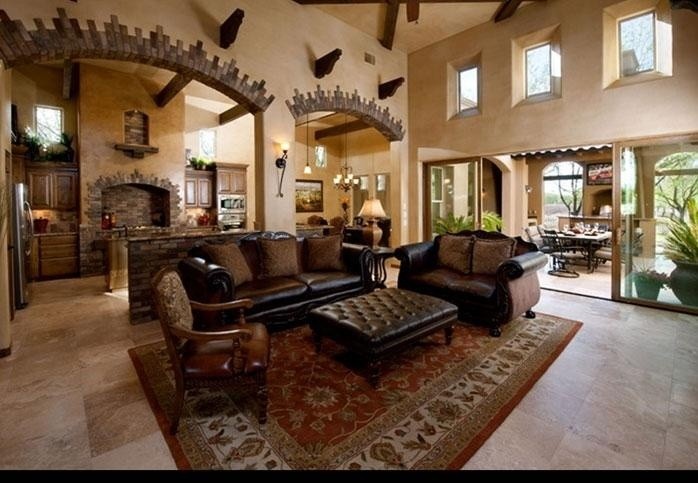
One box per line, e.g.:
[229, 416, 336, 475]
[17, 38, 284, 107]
[217, 194, 246, 229]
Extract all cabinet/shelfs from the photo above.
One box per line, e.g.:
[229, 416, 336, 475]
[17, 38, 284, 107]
[23, 162, 76, 209]
[184, 169, 216, 208]
[29, 234, 79, 277]
[354, 247, 395, 285]
[216, 162, 247, 192]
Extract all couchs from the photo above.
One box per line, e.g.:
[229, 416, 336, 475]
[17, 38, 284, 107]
[177, 230, 376, 335]
[392, 228, 547, 336]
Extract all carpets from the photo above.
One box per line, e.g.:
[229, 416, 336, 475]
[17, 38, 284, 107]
[128, 310, 585, 469]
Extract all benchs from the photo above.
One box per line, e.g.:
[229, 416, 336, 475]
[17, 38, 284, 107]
[306, 286, 457, 388]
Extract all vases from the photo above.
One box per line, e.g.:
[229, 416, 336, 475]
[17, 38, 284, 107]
[635, 281, 659, 300]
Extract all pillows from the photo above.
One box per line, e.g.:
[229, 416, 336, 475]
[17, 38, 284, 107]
[477, 238, 514, 278]
[306, 230, 343, 273]
[256, 234, 301, 277]
[199, 240, 253, 287]
[433, 232, 472, 276]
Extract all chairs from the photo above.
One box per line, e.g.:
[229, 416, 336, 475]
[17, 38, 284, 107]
[153, 268, 274, 432]
[523, 220, 625, 277]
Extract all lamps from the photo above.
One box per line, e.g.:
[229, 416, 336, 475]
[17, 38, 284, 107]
[275, 142, 289, 198]
[333, 113, 361, 192]
[356, 199, 386, 245]
[303, 111, 312, 174]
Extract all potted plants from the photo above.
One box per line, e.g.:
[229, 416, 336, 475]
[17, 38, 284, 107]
[658, 182, 697, 305]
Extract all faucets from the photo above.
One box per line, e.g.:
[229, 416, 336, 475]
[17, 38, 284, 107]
[122, 224, 128, 237]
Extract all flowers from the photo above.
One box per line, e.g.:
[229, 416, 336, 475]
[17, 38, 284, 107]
[630, 267, 669, 287]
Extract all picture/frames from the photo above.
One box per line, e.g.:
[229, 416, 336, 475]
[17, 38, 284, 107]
[296, 178, 324, 212]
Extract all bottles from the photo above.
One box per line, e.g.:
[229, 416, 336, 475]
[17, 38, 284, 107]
[111, 211, 116, 227]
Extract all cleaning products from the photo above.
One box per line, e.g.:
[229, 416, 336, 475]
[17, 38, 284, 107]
[102, 205, 111, 227]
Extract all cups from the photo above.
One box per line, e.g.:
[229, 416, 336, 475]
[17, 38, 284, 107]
[35, 218, 48, 232]
[563, 220, 599, 232]
[199, 215, 208, 225]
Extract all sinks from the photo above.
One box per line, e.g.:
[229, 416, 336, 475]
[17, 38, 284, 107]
[115, 234, 137, 237]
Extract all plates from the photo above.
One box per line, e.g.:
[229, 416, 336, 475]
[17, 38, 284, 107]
[584, 232, 594, 235]
[564, 233, 575, 236]
